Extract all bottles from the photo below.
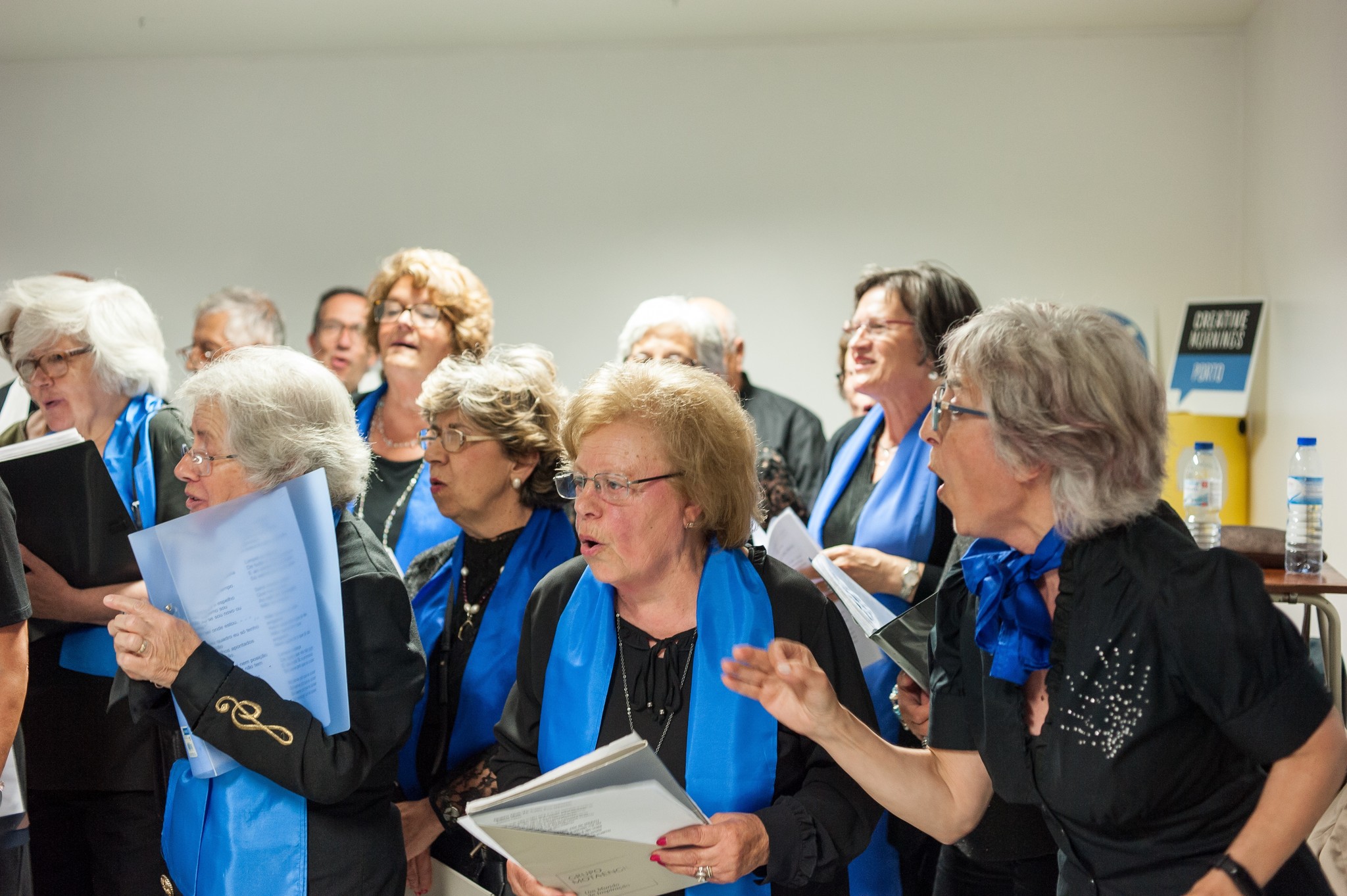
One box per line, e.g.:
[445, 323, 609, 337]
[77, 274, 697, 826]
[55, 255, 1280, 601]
[1285, 437, 1325, 575]
[1184, 440, 1222, 550]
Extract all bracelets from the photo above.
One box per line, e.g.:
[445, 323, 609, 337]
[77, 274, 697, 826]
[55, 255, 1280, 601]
[1211, 853, 1262, 896]
[886, 684, 909, 734]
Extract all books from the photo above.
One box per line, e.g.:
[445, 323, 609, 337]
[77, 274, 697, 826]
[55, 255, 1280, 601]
[0, 427, 141, 639]
[809, 546, 943, 697]
[466, 736, 715, 896]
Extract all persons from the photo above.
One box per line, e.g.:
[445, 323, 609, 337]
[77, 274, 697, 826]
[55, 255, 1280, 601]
[0, 247, 1056, 896]
[99, 346, 422, 896]
[488, 360, 890, 896]
[718, 299, 1347, 896]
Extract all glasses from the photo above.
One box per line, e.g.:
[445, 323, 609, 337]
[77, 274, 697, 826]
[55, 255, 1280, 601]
[373, 298, 451, 327]
[14, 344, 95, 382]
[932, 383, 996, 432]
[418, 427, 498, 452]
[178, 344, 229, 366]
[553, 464, 678, 503]
[841, 317, 921, 341]
[180, 441, 247, 477]
[0, 331, 22, 354]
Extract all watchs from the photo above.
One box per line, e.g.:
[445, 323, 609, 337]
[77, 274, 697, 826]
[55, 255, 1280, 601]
[899, 560, 921, 600]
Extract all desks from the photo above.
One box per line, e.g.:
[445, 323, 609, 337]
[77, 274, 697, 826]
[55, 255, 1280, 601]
[1263, 561, 1347, 723]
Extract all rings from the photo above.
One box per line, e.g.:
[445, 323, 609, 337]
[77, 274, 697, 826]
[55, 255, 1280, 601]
[133, 639, 148, 654]
[706, 866, 713, 879]
[921, 736, 928, 750]
[694, 867, 706, 884]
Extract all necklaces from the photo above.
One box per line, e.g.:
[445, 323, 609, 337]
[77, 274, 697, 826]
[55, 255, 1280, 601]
[375, 400, 417, 450]
[615, 608, 696, 754]
[878, 438, 896, 464]
[456, 553, 505, 644]
[358, 422, 423, 546]
